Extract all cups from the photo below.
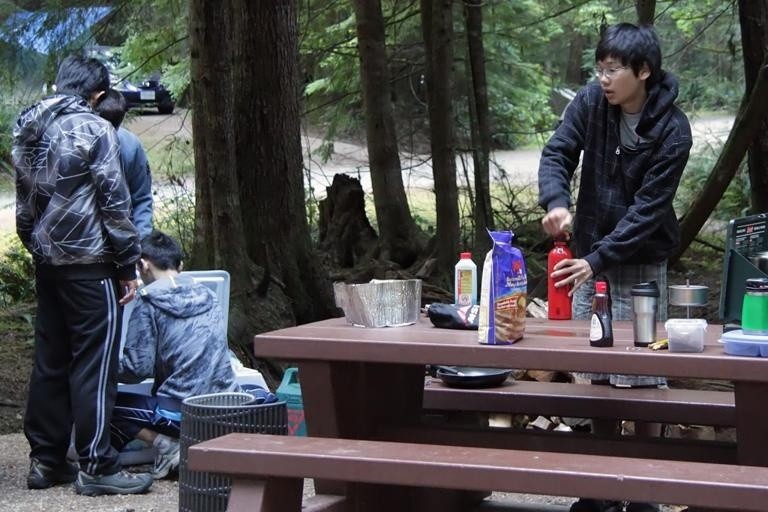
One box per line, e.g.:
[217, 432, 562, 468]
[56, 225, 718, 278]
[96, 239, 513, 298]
[628, 280, 660, 348]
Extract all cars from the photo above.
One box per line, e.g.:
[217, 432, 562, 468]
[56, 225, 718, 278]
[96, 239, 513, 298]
[56, 45, 175, 114]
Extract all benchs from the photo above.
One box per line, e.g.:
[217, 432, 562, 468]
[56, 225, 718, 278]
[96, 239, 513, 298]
[422, 375, 741, 429]
[186, 432, 768, 512]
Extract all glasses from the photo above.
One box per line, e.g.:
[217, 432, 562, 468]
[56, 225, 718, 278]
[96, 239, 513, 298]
[596, 66, 632, 79]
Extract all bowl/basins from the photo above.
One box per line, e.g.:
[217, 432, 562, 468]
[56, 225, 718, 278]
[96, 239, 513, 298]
[432, 365, 513, 389]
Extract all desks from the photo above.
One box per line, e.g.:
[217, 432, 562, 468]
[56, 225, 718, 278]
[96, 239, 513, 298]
[255, 317, 768, 512]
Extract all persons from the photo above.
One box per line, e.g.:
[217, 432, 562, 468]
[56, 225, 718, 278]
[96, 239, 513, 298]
[113, 228, 249, 479]
[92, 88, 156, 279]
[539, 21, 694, 511]
[7, 54, 152, 501]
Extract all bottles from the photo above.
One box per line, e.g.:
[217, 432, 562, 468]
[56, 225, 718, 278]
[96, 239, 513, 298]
[547, 232, 573, 321]
[587, 280, 614, 347]
[453, 252, 478, 306]
[741, 275, 768, 337]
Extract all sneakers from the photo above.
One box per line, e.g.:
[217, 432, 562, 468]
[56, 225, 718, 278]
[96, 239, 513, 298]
[570, 498, 659, 512]
[27, 433, 180, 496]
